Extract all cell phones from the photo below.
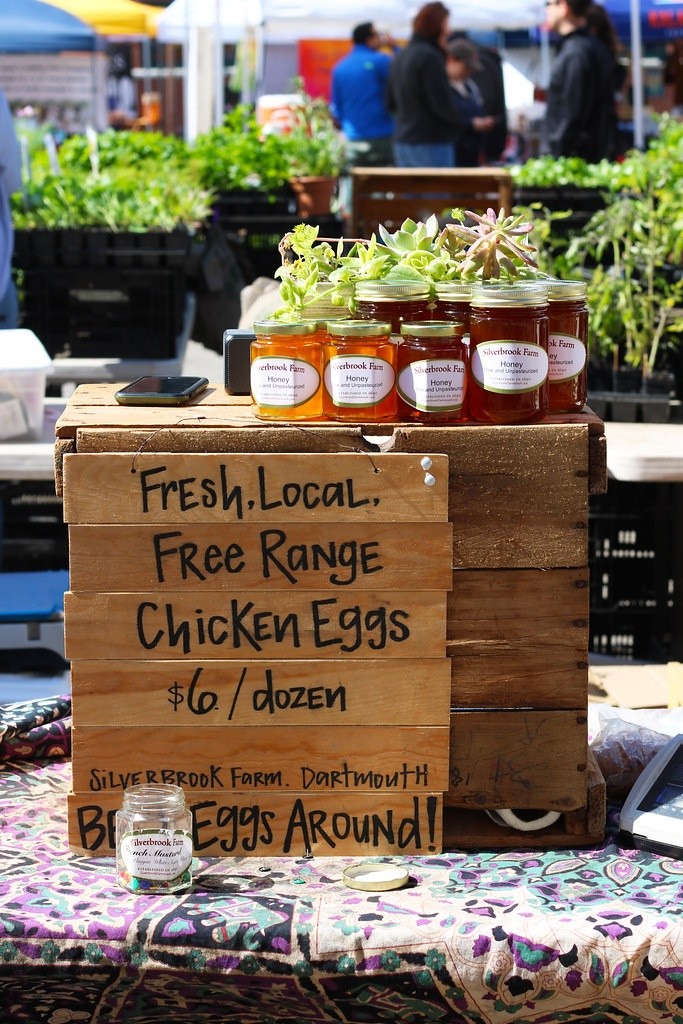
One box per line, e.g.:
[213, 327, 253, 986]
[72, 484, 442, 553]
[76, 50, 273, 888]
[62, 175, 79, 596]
[115, 374, 210, 404]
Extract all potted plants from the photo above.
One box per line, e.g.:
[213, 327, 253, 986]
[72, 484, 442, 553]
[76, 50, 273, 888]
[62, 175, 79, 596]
[508, 109, 683, 425]
[7, 127, 216, 363]
[193, 104, 350, 222]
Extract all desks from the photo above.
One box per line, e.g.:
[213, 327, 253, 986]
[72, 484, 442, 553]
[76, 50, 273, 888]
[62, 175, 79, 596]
[0, 693, 683, 1024]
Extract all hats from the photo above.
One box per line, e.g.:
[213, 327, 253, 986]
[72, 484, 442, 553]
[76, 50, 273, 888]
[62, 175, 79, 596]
[446, 38, 484, 72]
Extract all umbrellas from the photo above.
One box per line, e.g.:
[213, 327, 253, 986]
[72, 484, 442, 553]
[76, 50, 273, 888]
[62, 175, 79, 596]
[41, 0, 166, 39]
[0, 0, 109, 54]
[531, 0, 683, 43]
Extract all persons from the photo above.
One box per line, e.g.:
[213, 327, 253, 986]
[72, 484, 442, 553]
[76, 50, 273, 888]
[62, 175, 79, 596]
[0, 84, 23, 329]
[326, 1, 627, 199]
[107, 54, 137, 113]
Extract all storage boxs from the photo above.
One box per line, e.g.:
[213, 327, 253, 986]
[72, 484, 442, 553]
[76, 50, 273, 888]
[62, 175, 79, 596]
[56, 381, 608, 856]
[15, 284, 196, 363]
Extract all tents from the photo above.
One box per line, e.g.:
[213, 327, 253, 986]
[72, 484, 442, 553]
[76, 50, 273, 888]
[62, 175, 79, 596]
[158, 0, 553, 144]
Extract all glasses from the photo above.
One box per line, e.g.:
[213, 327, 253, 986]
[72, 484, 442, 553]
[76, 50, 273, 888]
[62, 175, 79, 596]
[545, 0, 557, 6]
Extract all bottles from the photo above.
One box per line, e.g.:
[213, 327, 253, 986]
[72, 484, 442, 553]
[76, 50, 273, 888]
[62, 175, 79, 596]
[114, 783, 193, 895]
